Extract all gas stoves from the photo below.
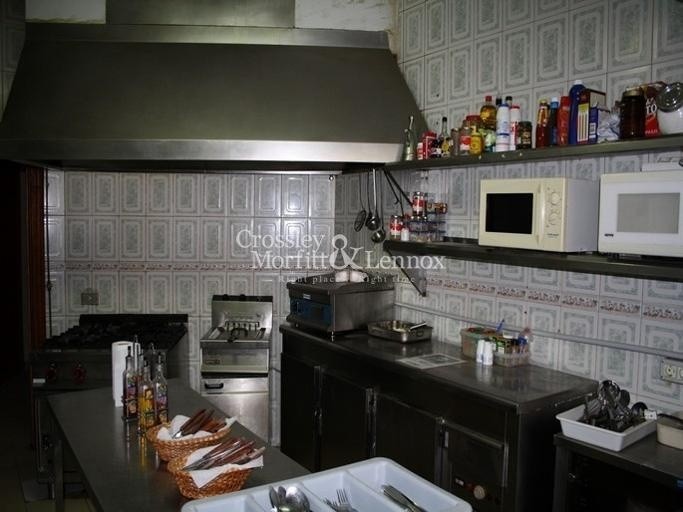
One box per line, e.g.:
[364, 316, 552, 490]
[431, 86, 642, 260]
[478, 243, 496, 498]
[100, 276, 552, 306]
[40, 323, 187, 350]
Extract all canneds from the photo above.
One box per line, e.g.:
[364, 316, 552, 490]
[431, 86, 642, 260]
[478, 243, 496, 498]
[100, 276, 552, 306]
[518, 120, 534, 148]
[470, 134, 483, 155]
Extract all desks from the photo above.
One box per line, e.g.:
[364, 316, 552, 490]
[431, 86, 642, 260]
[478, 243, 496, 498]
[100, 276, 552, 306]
[48, 376, 313, 511]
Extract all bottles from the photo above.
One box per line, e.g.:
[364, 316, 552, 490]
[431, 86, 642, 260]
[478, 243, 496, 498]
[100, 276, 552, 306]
[403, 116, 416, 161]
[458, 120, 471, 156]
[137, 360, 154, 434]
[510, 338, 527, 366]
[417, 142, 423, 160]
[536, 80, 586, 148]
[476, 340, 494, 365]
[481, 95, 522, 152]
[153, 355, 169, 425]
[438, 117, 450, 157]
[389, 191, 428, 244]
[122, 345, 138, 419]
[619, 90, 647, 139]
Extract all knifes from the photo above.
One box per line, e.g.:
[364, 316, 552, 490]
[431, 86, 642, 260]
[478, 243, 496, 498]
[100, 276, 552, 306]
[384, 485, 427, 512]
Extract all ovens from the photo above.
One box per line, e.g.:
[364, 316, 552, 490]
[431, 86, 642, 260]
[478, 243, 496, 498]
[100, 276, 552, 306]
[440, 421, 510, 512]
[285, 274, 395, 332]
[34, 390, 92, 474]
[200, 377, 270, 444]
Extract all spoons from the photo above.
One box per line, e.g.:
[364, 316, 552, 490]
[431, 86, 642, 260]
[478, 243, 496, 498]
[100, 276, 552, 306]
[269, 485, 315, 512]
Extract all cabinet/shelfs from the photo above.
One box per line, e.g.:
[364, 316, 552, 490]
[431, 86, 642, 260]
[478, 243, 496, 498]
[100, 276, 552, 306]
[554, 432, 682, 512]
[384, 99, 682, 297]
[279, 321, 600, 512]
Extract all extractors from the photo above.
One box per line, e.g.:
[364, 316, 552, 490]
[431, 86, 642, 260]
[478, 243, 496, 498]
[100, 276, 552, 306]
[1, 2, 429, 176]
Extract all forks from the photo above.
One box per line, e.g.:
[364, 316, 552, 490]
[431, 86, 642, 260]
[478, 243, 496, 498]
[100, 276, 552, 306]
[336, 489, 356, 512]
[323, 498, 339, 512]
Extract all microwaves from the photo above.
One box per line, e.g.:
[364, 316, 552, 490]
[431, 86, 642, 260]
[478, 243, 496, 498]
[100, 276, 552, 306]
[477, 178, 599, 252]
[598, 171, 683, 258]
